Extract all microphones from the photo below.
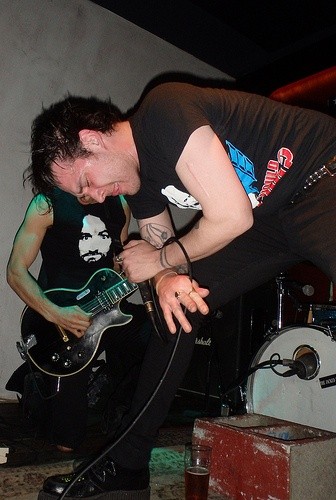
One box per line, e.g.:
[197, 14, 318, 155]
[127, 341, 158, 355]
[292, 351, 317, 378]
[125, 233, 154, 312]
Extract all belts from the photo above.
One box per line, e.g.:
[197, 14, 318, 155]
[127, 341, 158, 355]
[285, 154, 336, 208]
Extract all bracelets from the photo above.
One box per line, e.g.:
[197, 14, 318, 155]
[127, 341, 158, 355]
[154, 270, 178, 298]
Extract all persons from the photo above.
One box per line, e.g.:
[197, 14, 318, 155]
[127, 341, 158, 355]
[29, 80, 336, 500]
[8, 184, 146, 451]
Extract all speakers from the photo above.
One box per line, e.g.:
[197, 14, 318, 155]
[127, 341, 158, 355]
[176, 283, 276, 405]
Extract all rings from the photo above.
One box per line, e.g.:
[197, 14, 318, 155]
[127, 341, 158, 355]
[114, 254, 122, 263]
[174, 291, 183, 299]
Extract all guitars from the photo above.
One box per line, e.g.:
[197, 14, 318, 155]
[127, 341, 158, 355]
[19, 267, 138, 378]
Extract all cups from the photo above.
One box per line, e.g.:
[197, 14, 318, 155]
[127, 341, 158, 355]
[184, 444, 213, 500]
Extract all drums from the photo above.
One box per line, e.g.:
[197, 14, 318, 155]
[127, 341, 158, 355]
[244, 324, 336, 433]
[293, 301, 336, 332]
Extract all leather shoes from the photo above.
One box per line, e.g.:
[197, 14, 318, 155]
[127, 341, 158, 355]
[37, 455, 151, 500]
